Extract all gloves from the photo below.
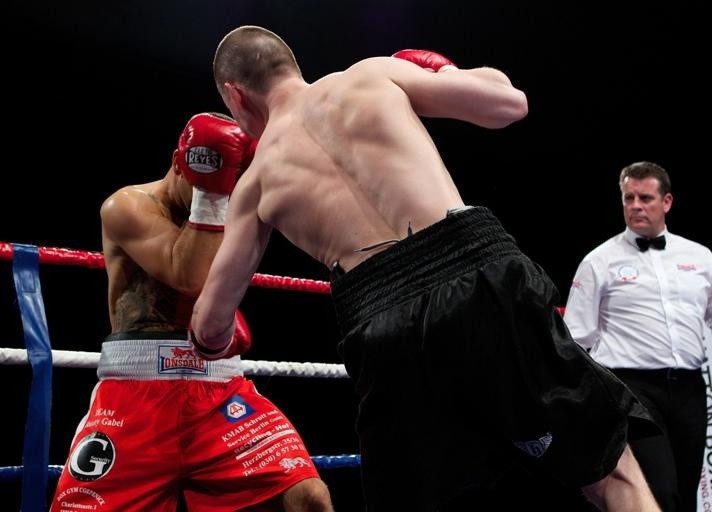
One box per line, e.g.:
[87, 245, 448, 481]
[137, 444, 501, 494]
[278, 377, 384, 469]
[178, 112, 258, 232]
[391, 50, 458, 73]
[188, 307, 252, 362]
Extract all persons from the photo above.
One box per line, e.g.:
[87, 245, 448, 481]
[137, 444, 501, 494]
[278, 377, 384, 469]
[187, 25, 663, 511]
[48, 110, 335, 510]
[562, 162, 711, 512]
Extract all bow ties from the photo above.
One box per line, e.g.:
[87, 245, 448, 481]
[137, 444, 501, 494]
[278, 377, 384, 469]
[635, 235, 665, 252]
